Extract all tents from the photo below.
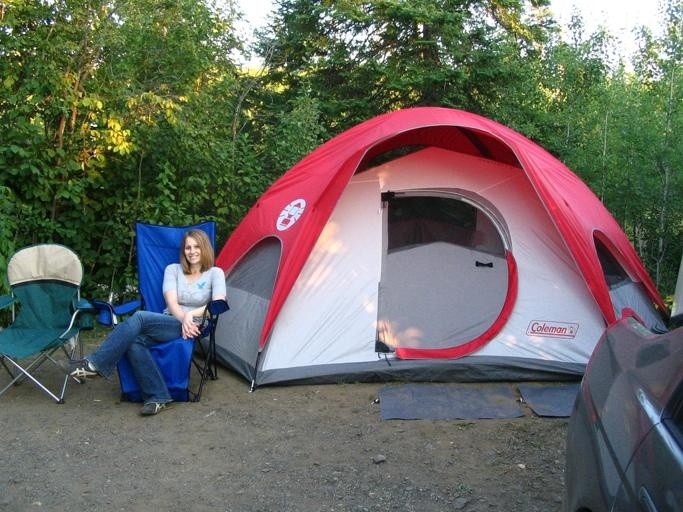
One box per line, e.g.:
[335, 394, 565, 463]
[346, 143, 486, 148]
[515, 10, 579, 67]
[197, 104, 673, 397]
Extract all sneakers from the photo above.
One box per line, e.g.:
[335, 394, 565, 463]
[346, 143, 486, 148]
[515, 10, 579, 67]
[140, 401, 173, 415]
[68, 360, 98, 380]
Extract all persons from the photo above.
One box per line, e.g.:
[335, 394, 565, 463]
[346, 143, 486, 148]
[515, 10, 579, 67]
[54, 228, 227, 417]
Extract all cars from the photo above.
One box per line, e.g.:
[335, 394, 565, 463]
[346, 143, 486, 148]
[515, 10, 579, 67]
[556, 316, 681, 511]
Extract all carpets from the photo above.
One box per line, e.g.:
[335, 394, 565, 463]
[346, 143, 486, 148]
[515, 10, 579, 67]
[375, 381, 579, 422]
